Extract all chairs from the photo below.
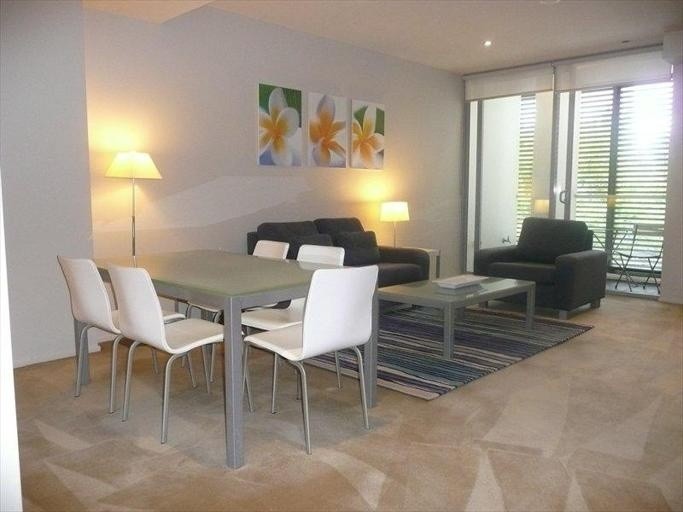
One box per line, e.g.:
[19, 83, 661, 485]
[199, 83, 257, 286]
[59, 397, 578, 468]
[181, 240, 292, 385]
[243, 244, 347, 402]
[242, 267, 379, 455]
[614, 223, 664, 294]
[474, 224, 607, 320]
[56, 255, 158, 414]
[107, 262, 257, 446]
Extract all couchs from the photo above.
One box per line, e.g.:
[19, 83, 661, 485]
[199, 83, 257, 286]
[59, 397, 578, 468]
[246, 230, 429, 311]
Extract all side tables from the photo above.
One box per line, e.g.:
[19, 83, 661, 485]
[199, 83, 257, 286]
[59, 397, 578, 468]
[387, 244, 441, 278]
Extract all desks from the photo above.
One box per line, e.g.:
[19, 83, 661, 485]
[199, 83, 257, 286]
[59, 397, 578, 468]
[71, 250, 380, 469]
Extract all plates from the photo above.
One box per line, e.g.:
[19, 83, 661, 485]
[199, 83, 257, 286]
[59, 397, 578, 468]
[432, 274, 488, 289]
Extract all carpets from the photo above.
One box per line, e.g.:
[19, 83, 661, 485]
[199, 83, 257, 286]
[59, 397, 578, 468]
[304, 303, 595, 401]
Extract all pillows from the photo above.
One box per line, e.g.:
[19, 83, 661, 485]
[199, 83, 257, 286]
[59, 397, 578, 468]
[516, 217, 587, 262]
[258, 218, 381, 266]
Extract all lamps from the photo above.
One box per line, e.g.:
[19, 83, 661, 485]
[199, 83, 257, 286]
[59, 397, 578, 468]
[380, 200, 410, 249]
[105, 151, 163, 259]
